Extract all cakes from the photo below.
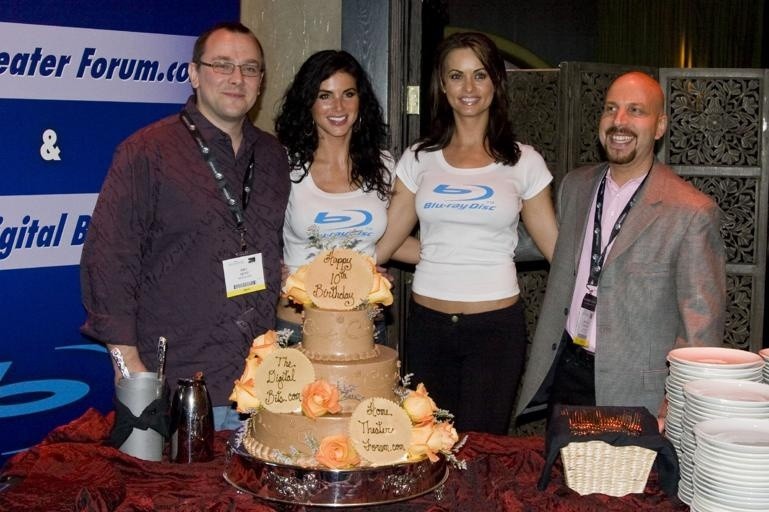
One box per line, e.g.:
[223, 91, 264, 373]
[229, 250, 458, 468]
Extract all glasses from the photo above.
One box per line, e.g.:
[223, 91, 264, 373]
[198, 59, 263, 77]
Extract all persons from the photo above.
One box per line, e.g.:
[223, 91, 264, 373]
[271, 49, 421, 348]
[521, 71, 728, 432]
[371, 29, 559, 436]
[78, 22, 292, 431]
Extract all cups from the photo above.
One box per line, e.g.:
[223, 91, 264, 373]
[111, 371, 167, 463]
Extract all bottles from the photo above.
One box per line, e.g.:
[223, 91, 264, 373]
[170, 377, 215, 464]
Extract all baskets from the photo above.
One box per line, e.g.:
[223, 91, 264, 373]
[554, 405, 658, 498]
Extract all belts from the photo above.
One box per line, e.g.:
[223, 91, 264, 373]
[559, 336, 593, 372]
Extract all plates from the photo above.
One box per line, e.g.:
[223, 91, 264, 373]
[663, 347, 769, 511]
